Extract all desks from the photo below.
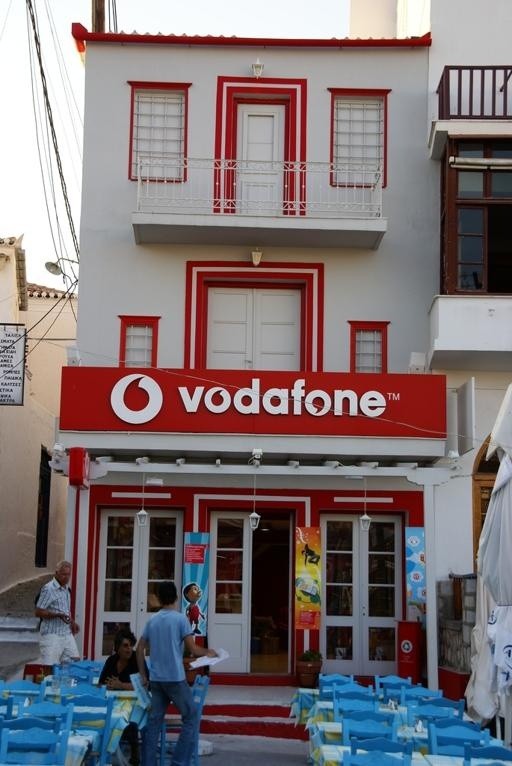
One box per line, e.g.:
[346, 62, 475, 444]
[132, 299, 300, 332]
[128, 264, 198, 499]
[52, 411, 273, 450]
[291, 687, 384, 729]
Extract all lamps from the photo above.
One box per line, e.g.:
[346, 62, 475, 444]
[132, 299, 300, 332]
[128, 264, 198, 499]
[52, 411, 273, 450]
[250, 246, 263, 266]
[251, 57, 265, 78]
[360, 477, 372, 531]
[248, 473, 262, 529]
[136, 473, 149, 526]
[44, 257, 80, 275]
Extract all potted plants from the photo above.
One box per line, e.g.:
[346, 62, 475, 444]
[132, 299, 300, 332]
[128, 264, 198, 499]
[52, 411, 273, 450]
[297, 649, 323, 686]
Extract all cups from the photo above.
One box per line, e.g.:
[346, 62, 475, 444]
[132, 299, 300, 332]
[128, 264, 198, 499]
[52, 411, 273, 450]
[52, 675, 61, 691]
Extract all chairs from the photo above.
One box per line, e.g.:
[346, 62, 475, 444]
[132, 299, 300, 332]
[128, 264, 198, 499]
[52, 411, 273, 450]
[0, 659, 211, 766]
[314, 673, 512, 765]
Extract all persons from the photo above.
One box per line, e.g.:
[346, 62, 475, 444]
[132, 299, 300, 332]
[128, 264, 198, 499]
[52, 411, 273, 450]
[97, 631, 151, 766]
[35, 561, 80, 676]
[136, 582, 219, 766]
[183, 583, 205, 634]
